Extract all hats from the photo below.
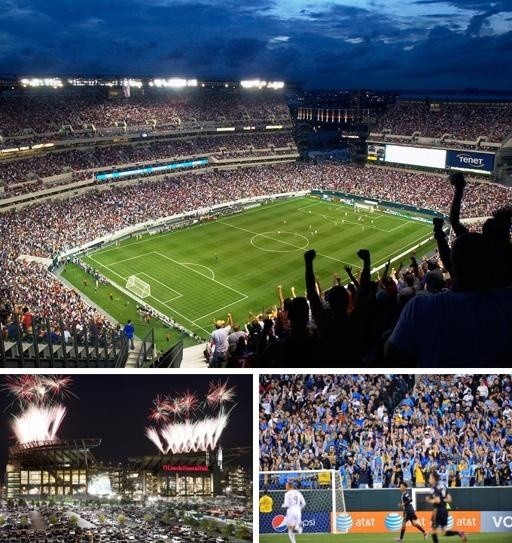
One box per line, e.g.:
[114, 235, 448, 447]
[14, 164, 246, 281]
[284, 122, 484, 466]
[216, 320, 225, 326]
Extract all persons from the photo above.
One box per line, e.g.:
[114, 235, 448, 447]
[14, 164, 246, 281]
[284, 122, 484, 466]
[1, 87, 512, 368]
[259, 374, 511, 541]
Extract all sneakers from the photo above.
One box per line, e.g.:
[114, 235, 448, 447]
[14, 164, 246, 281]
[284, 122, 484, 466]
[393, 531, 465, 542]
